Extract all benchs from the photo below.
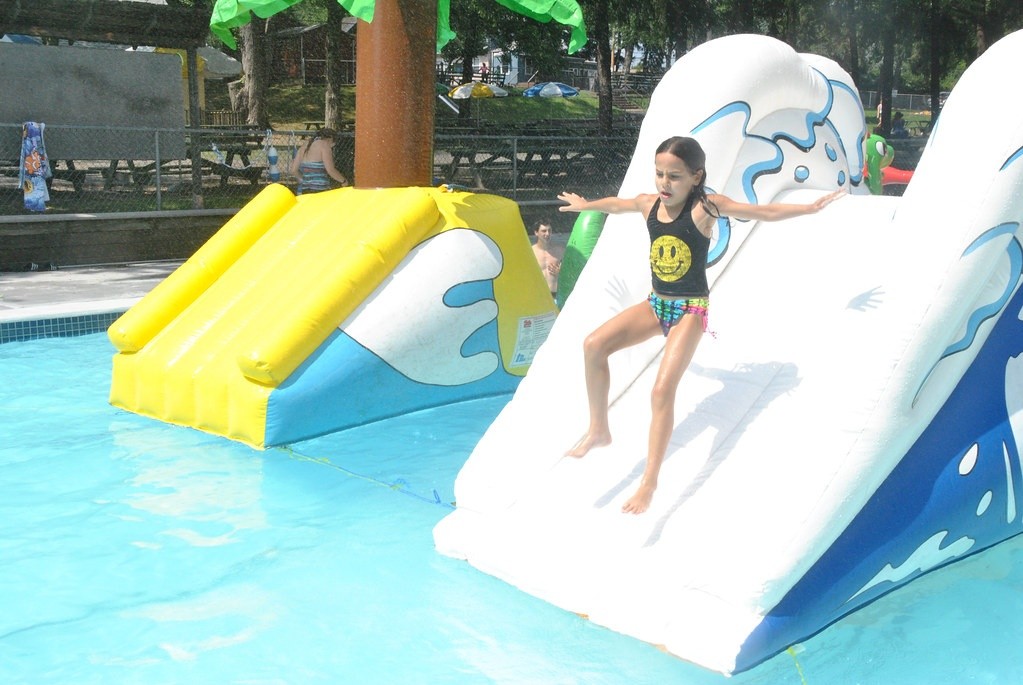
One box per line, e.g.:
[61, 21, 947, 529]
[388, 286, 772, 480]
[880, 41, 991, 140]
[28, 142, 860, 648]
[4, 119, 642, 202]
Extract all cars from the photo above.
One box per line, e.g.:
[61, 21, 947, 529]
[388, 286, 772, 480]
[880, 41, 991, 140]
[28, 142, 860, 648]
[927, 91, 951, 109]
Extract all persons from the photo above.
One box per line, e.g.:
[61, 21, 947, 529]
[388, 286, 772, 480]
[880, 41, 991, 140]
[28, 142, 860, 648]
[532, 217, 564, 299]
[291, 128, 348, 194]
[556, 138, 846, 513]
[479, 63, 501, 82]
[876, 99, 882, 127]
[892, 111, 905, 131]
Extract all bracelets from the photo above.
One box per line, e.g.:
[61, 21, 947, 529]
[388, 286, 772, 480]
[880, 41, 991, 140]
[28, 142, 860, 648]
[340, 179, 347, 184]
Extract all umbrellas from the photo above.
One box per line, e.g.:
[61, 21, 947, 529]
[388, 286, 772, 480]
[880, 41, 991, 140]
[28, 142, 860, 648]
[448, 82, 509, 127]
[522, 81, 579, 129]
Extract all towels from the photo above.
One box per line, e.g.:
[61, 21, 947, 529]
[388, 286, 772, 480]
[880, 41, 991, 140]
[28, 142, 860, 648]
[18, 121, 52, 212]
